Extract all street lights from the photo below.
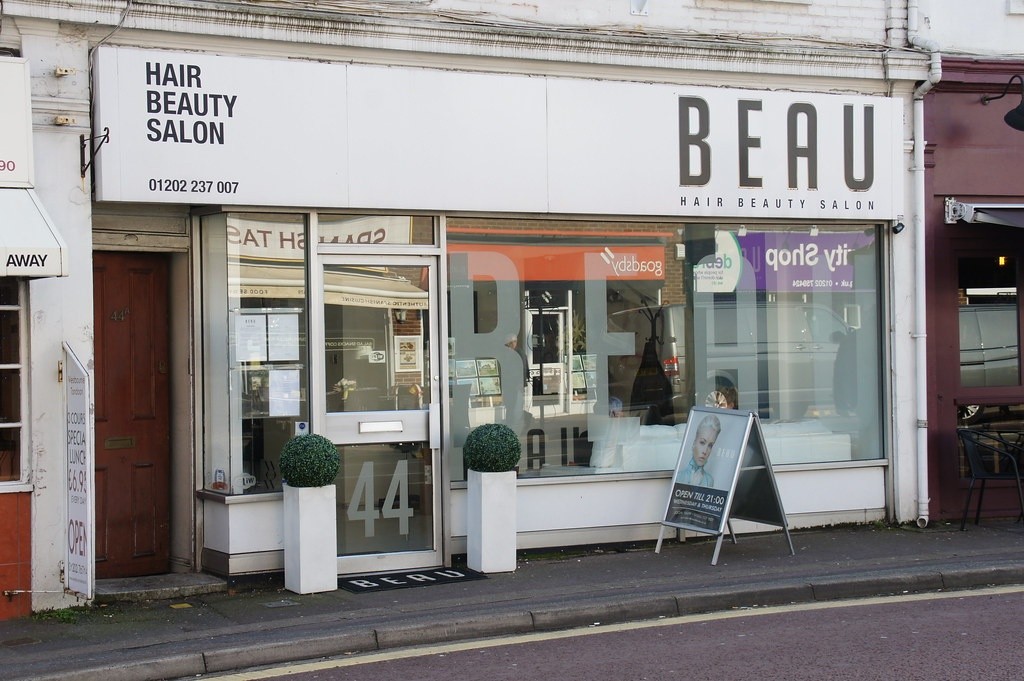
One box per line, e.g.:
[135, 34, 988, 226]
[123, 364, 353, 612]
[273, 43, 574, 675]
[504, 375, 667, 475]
[522, 292, 561, 441]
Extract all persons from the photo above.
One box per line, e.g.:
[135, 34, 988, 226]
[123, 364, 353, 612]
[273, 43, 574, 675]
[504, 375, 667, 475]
[543, 352, 554, 364]
[498, 332, 529, 426]
[710, 385, 737, 410]
[575, 397, 624, 469]
[606, 279, 668, 408]
[675, 414, 722, 488]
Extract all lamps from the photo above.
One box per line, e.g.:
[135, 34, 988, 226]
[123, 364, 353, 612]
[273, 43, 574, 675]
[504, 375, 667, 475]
[982, 75, 1024, 131]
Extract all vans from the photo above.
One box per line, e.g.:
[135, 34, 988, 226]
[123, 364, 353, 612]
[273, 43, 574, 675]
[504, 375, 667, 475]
[958, 303, 1019, 425]
[594, 302, 859, 425]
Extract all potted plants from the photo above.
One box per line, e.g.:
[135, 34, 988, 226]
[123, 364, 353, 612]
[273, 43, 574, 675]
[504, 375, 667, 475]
[279, 433, 341, 595]
[463, 424, 522, 573]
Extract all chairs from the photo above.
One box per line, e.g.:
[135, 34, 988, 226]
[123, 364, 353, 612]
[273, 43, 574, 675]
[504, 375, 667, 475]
[958, 429, 1024, 532]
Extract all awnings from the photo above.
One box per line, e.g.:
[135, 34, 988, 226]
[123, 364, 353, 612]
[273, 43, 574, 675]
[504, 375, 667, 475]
[226, 260, 430, 310]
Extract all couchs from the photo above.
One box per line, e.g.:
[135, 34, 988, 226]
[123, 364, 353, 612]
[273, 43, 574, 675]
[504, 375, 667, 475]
[542, 417, 853, 475]
